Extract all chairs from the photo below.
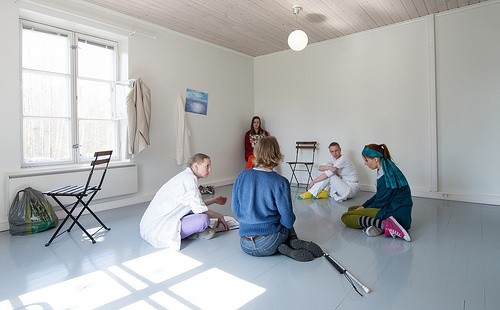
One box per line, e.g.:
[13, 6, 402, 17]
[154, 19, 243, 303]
[286, 142, 317, 190]
[42, 151, 113, 247]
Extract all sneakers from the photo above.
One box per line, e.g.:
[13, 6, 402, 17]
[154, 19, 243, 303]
[383, 215, 411, 242]
[384, 239, 410, 256]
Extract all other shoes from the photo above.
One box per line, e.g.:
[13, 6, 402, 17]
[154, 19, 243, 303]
[198, 185, 215, 195]
[366, 225, 383, 236]
[278, 244, 314, 262]
[290, 240, 323, 257]
[188, 228, 216, 240]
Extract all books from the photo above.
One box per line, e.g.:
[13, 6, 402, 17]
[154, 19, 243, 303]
[208, 215, 240, 232]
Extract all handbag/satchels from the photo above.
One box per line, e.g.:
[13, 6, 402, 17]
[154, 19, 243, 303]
[8, 187, 59, 236]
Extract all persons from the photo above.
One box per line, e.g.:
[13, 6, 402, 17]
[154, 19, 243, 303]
[341, 144, 413, 241]
[297, 142, 359, 203]
[244, 116, 270, 169]
[139, 153, 229, 250]
[231, 136, 323, 262]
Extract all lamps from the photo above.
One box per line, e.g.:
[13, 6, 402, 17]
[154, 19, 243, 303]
[288, 6, 308, 51]
